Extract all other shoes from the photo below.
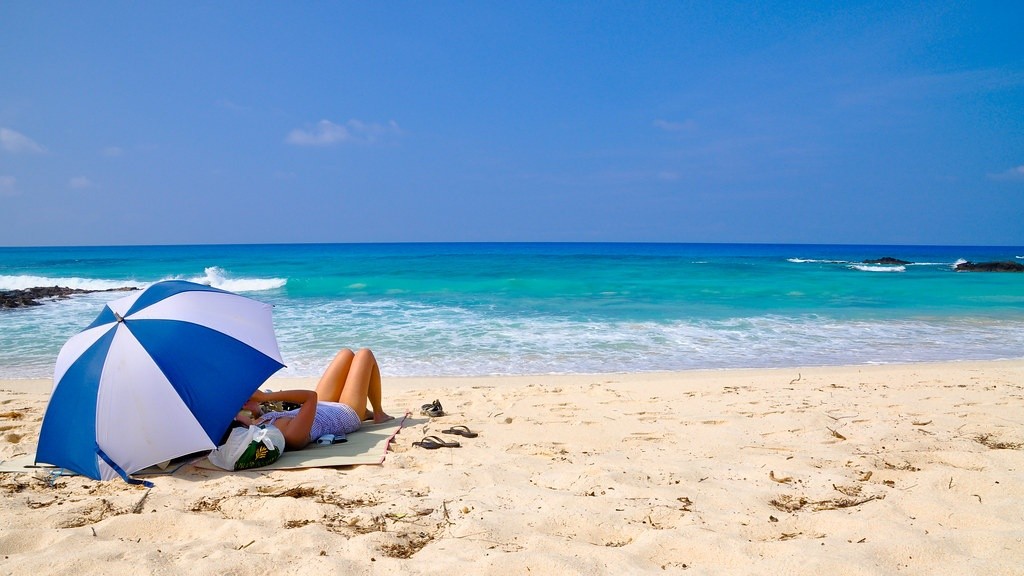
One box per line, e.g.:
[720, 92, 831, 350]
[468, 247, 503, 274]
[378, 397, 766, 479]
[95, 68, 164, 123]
[421, 399, 444, 416]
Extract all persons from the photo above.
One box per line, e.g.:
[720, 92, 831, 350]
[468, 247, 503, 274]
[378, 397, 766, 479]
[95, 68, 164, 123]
[221, 348, 396, 452]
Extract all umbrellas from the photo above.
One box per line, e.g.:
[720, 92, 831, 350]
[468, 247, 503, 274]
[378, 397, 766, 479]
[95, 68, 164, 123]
[35, 280, 286, 488]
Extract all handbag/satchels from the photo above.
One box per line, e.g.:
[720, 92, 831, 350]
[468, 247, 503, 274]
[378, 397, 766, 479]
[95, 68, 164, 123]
[208, 425, 285, 471]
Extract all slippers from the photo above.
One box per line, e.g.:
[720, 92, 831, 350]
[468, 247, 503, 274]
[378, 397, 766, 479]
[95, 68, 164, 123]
[442, 425, 478, 438]
[412, 435, 460, 449]
